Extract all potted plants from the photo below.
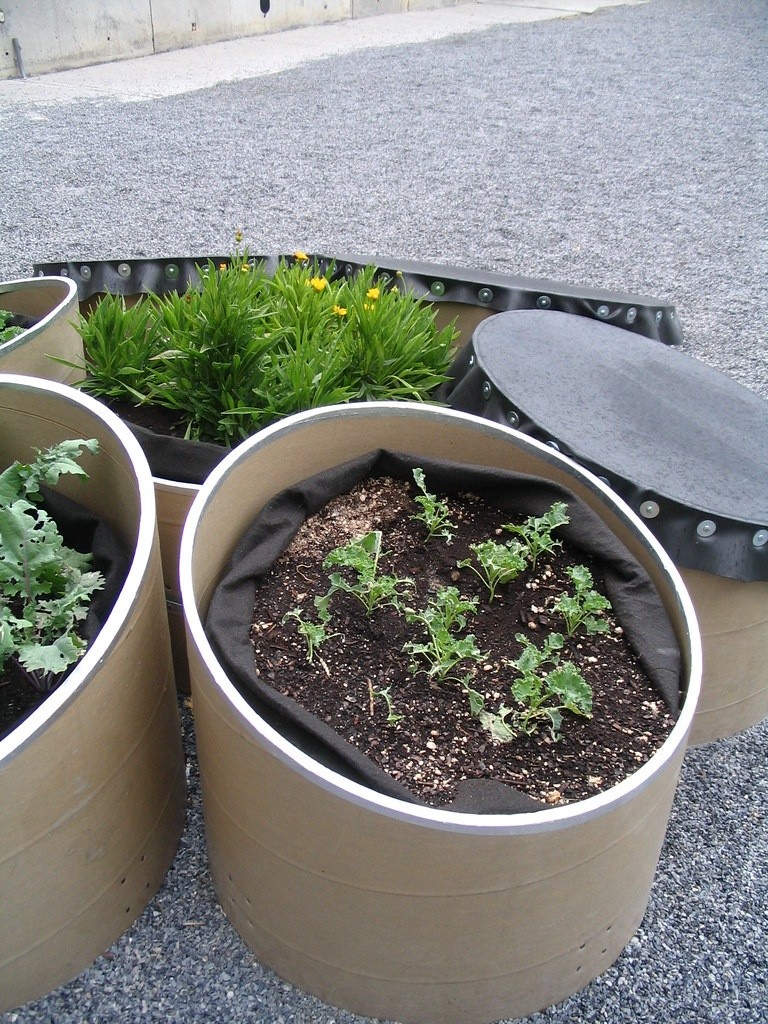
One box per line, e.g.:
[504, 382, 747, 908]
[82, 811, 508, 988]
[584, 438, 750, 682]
[178, 398, 705, 1024]
[0, 276, 89, 398]
[0, 372, 188, 1009]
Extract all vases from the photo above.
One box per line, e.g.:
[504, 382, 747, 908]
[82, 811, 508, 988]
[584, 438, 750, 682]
[64, 412, 460, 692]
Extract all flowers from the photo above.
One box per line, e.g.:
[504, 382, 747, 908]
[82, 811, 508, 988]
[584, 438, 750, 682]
[75, 249, 466, 444]
